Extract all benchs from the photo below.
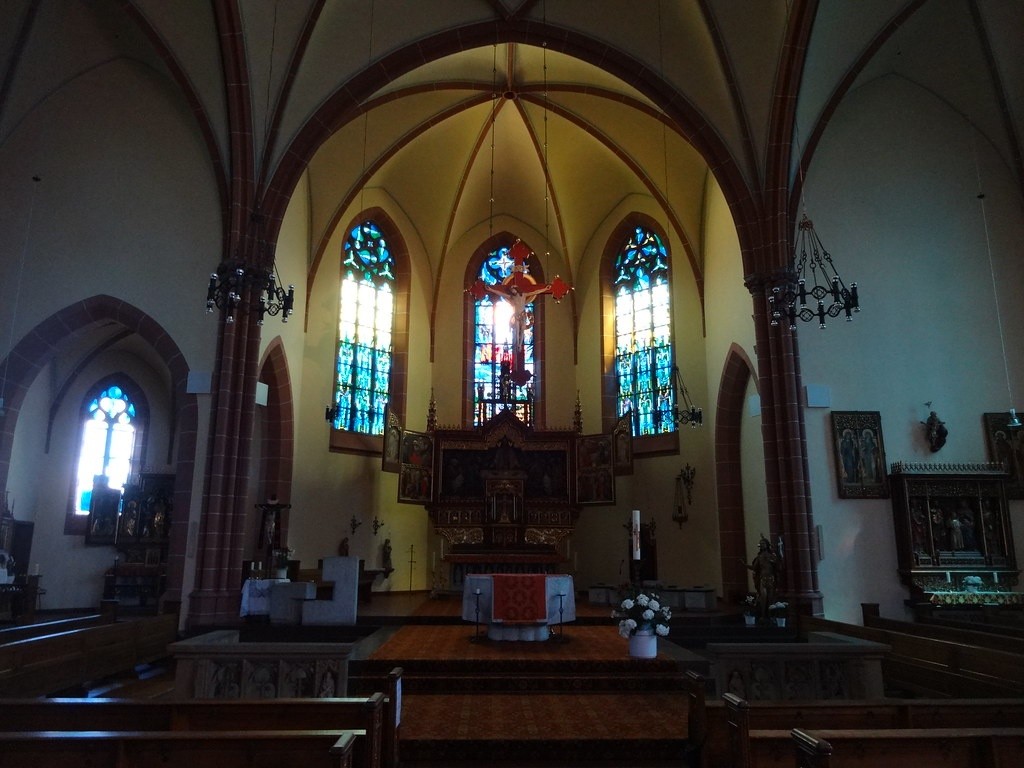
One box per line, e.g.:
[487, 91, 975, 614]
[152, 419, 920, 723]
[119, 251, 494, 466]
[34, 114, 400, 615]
[685, 666, 1024, 768]
[0, 599, 183, 698]
[0, 667, 404, 768]
[860, 597, 1023, 655]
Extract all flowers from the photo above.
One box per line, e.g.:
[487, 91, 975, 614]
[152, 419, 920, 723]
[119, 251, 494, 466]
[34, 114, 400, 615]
[768, 601, 788, 618]
[611, 591, 673, 639]
[7, 556, 16, 576]
[0, 549, 9, 569]
[962, 576, 982, 589]
[743, 595, 756, 617]
[273, 545, 296, 570]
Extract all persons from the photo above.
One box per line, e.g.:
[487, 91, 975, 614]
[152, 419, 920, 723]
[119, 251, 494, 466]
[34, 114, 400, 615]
[123, 501, 136, 536]
[384, 538, 392, 578]
[485, 285, 551, 352]
[737, 539, 780, 621]
[910, 492, 964, 553]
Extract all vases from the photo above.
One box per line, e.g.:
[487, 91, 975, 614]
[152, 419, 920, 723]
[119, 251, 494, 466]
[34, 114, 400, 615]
[630, 628, 658, 659]
[776, 617, 786, 628]
[275, 566, 288, 578]
[7, 576, 15, 584]
[0, 567, 7, 584]
[744, 613, 755, 625]
[966, 584, 977, 591]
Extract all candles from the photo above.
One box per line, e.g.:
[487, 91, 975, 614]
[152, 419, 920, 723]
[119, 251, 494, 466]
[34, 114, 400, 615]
[259, 561, 262, 570]
[632, 510, 641, 559]
[560, 590, 564, 595]
[35, 562, 39, 576]
[251, 561, 254, 570]
[476, 588, 481, 594]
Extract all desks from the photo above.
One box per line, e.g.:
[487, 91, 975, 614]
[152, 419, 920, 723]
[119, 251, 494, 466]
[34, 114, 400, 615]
[462, 575, 576, 642]
[240, 579, 290, 622]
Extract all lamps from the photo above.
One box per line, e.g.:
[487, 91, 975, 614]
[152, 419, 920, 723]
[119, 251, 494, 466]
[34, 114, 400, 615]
[204, 0, 295, 327]
[350, 514, 362, 536]
[765, 118, 862, 331]
[0, 175, 44, 416]
[976, 192, 1024, 431]
[373, 516, 384, 536]
[650, 0, 705, 431]
[327, 0, 378, 428]
[678, 462, 696, 506]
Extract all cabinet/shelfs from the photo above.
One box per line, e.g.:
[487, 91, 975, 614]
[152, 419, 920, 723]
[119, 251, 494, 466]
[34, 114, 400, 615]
[0, 516, 34, 585]
